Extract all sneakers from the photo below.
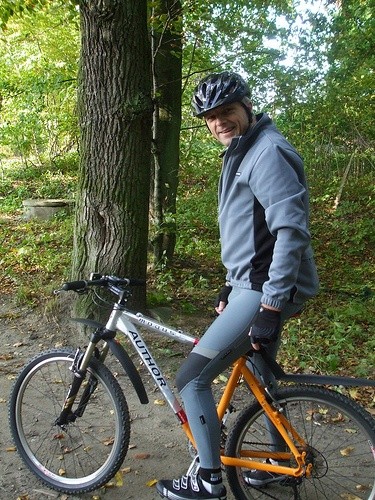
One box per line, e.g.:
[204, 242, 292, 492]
[241, 456, 291, 485]
[155, 455, 227, 500]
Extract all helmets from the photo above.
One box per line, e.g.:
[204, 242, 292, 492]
[191, 72, 251, 118]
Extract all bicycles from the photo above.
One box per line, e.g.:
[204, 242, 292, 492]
[8, 272, 375, 500]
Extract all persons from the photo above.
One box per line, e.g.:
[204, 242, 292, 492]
[155, 71, 320, 500]
